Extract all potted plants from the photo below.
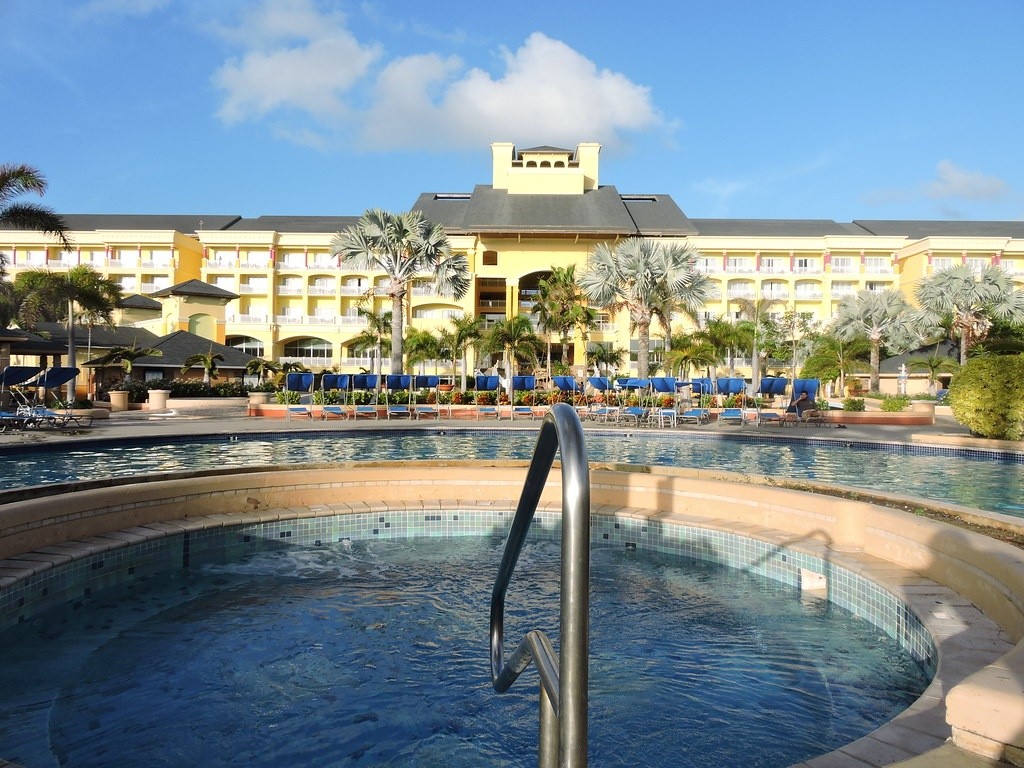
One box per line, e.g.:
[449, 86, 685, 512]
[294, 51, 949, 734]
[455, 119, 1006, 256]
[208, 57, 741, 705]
[436, 383, 455, 391]
[848, 384, 869, 397]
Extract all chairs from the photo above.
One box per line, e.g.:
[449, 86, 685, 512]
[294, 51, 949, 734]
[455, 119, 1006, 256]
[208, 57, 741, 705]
[352, 374, 379, 420]
[551, 376, 748, 428]
[752, 377, 788, 429]
[473, 375, 503, 420]
[786, 378, 832, 428]
[510, 376, 536, 419]
[321, 374, 349, 421]
[0, 366, 93, 432]
[414, 375, 440, 420]
[284, 373, 314, 422]
[386, 374, 412, 419]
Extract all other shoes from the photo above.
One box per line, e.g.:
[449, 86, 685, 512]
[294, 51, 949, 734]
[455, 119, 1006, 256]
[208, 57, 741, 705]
[835, 423, 847, 429]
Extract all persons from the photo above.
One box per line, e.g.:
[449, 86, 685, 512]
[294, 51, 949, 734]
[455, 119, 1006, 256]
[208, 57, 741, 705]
[791, 391, 824, 417]
